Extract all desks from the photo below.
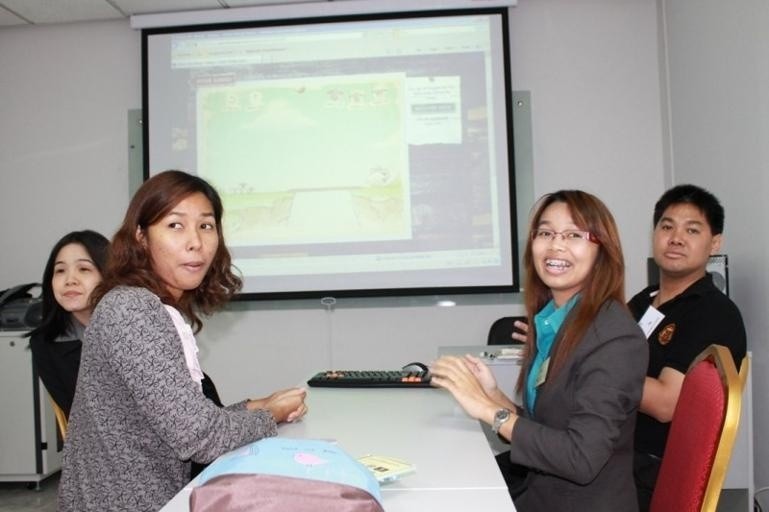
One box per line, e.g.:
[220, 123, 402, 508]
[156, 368, 517, 510]
[436, 347, 757, 512]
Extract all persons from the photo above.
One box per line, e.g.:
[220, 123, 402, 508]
[54, 168, 308, 511]
[425, 188, 642, 511]
[20, 229, 227, 484]
[495, 181, 749, 511]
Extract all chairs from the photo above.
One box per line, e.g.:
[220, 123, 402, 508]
[646, 343, 741, 512]
[46, 392, 69, 446]
[736, 351, 751, 391]
[487, 317, 530, 344]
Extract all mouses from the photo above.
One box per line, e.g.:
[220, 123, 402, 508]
[403, 362, 428, 374]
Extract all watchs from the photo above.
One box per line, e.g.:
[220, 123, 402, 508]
[490, 407, 513, 434]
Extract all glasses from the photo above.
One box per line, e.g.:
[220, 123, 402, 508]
[531, 228, 595, 243]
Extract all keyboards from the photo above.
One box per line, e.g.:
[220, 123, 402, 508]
[308, 372, 440, 389]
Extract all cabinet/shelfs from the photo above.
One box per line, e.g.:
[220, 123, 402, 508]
[0, 326, 68, 491]
